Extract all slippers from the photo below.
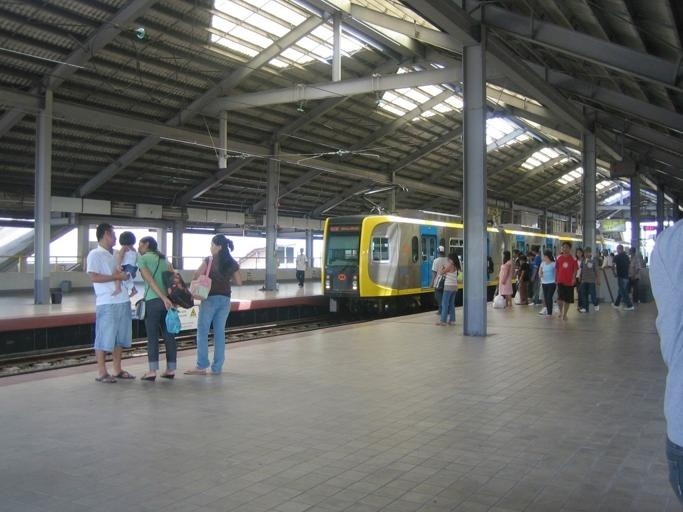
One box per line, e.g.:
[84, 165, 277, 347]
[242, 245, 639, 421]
[110, 369, 136, 381]
[93, 371, 117, 384]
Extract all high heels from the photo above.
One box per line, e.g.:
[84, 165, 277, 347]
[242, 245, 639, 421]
[140, 371, 156, 383]
[159, 370, 175, 380]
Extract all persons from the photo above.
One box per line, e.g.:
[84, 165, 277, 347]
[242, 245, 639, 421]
[487, 241, 647, 322]
[137, 236, 182, 382]
[648, 193, 683, 508]
[112, 232, 139, 299]
[86, 222, 138, 384]
[431, 247, 450, 316]
[296, 247, 307, 288]
[436, 253, 462, 328]
[185, 234, 242, 374]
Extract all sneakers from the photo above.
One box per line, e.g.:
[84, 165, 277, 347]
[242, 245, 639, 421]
[538, 306, 548, 315]
[578, 301, 640, 313]
[182, 366, 208, 376]
[436, 319, 456, 327]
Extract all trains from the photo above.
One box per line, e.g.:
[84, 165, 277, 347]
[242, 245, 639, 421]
[316, 207, 631, 320]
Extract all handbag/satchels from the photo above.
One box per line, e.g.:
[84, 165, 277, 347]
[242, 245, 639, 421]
[189, 254, 215, 303]
[160, 258, 195, 310]
[165, 306, 182, 335]
[492, 295, 508, 309]
[133, 298, 146, 322]
[433, 273, 446, 290]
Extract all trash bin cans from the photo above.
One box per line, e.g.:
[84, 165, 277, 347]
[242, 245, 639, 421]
[50, 288, 62, 304]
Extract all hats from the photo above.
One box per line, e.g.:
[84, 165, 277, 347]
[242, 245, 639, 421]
[435, 245, 445, 253]
